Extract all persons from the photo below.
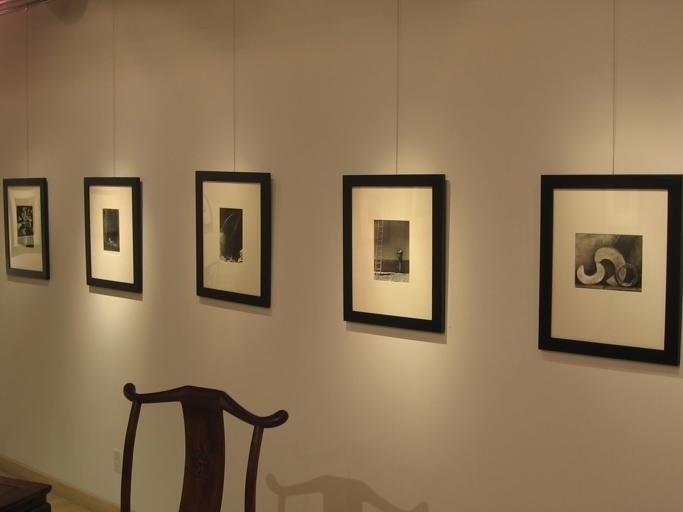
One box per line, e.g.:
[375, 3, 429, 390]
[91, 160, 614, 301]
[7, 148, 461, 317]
[396, 249, 403, 272]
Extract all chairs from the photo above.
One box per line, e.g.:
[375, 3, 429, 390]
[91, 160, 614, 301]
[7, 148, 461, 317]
[113, 380, 292, 512]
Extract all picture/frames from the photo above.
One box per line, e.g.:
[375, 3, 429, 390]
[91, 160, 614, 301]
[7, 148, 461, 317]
[340, 169, 449, 337]
[534, 172, 683, 371]
[0, 176, 144, 297]
[193, 170, 274, 310]
